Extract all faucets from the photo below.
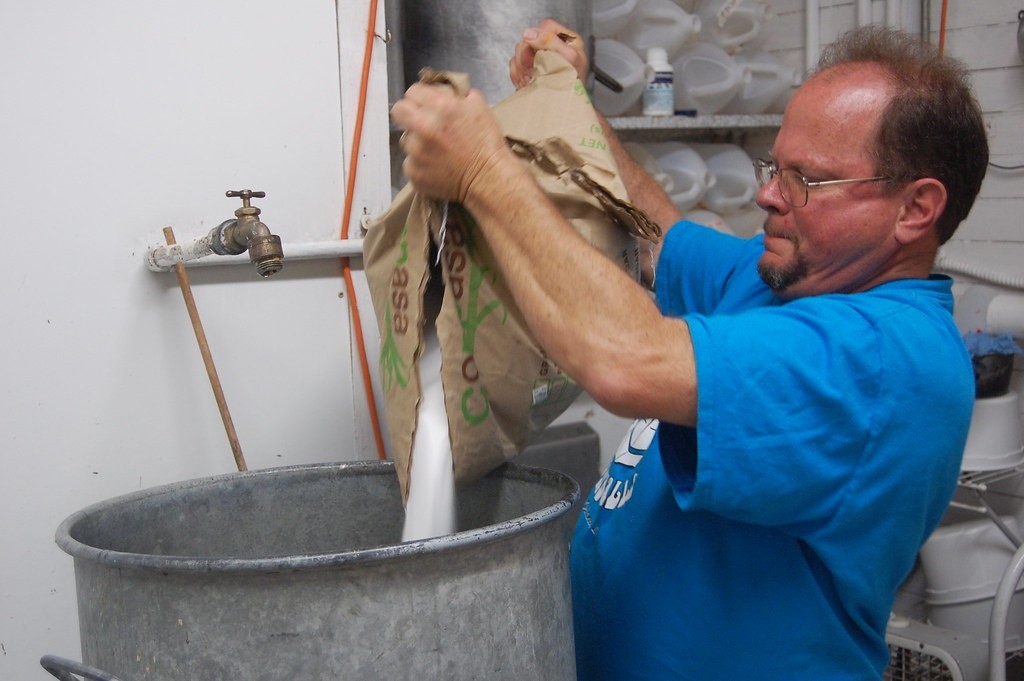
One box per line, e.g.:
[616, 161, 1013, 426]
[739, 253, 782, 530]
[204, 188, 287, 278]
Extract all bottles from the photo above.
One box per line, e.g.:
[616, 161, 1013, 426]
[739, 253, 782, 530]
[591, 1, 801, 238]
[642, 48, 674, 115]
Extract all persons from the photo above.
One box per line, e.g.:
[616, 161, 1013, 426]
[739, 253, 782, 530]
[391, 19, 990, 681]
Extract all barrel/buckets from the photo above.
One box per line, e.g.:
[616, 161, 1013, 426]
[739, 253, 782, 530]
[920, 516, 1024, 652]
[58, 461, 578, 681]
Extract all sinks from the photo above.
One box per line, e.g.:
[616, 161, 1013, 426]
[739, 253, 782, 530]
[53, 459, 584, 681]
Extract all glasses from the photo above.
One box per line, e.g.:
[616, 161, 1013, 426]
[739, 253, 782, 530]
[752, 156, 929, 208]
